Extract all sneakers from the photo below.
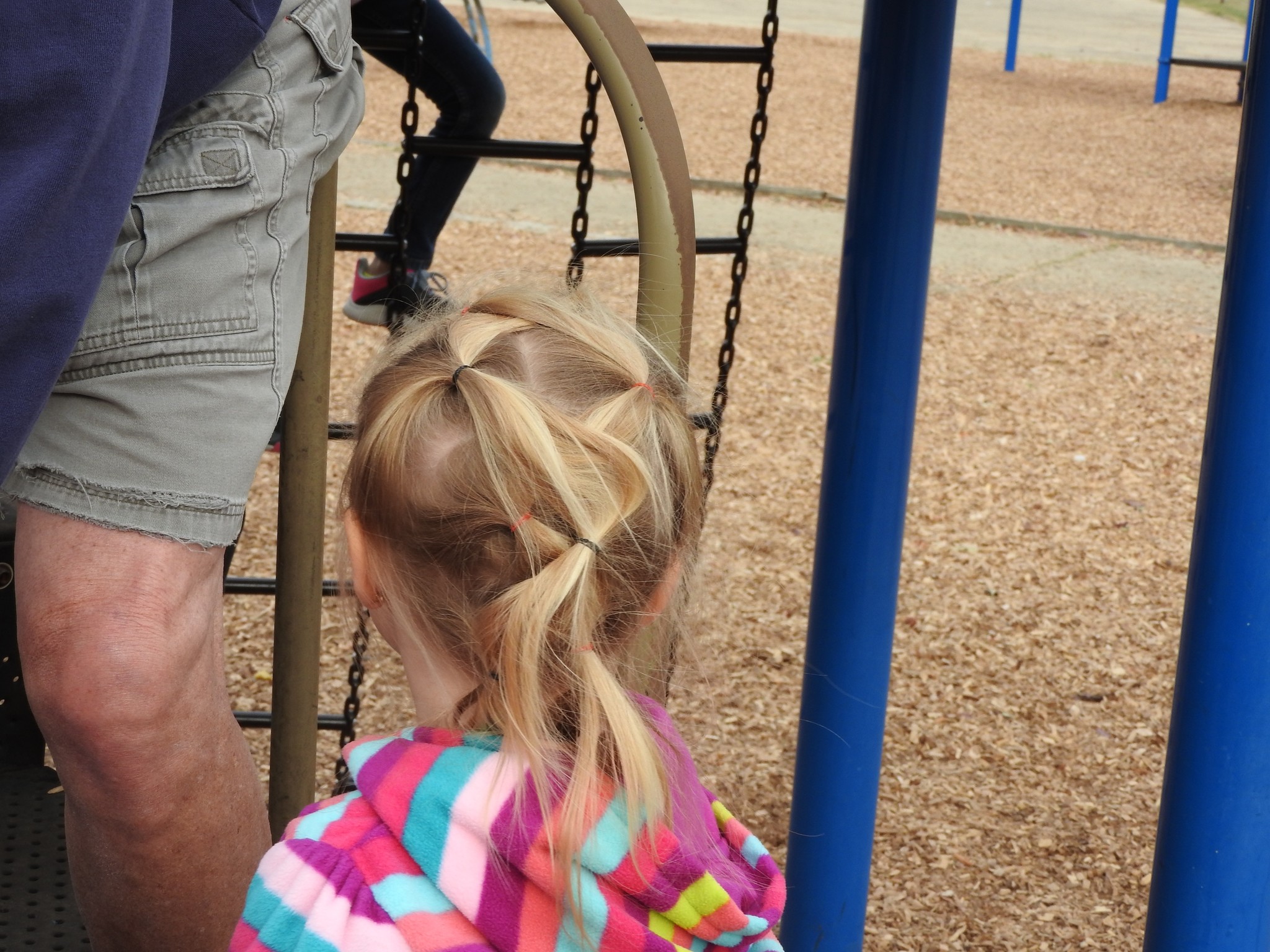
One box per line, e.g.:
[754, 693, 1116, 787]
[266, 433, 282, 451]
[342, 259, 462, 329]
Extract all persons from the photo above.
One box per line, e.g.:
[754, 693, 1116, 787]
[341, 0, 506, 329]
[2, 1, 365, 952]
[228, 287, 787, 952]
